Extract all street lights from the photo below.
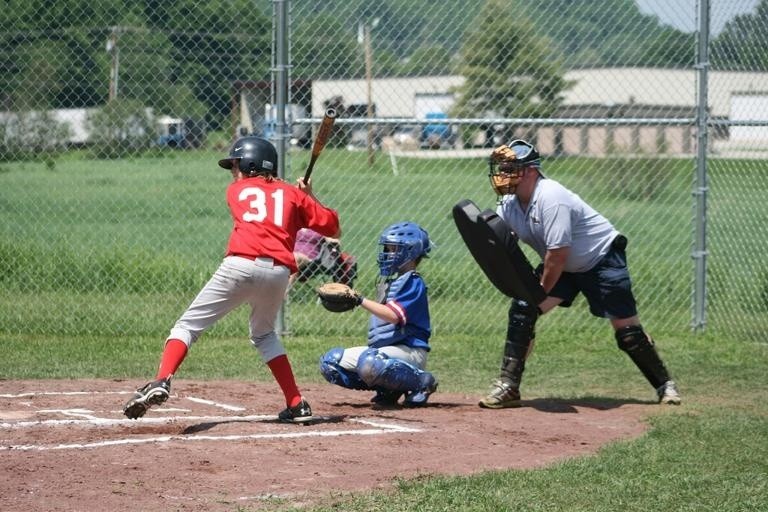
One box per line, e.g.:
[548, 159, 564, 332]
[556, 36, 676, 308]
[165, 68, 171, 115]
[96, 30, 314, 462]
[106, 24, 129, 103]
[358, 16, 382, 164]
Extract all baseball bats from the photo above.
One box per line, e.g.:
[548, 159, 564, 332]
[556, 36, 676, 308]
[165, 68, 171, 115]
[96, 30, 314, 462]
[305, 108, 337, 183]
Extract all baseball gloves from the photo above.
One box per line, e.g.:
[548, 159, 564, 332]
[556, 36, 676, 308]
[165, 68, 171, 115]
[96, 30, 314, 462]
[318, 284, 357, 311]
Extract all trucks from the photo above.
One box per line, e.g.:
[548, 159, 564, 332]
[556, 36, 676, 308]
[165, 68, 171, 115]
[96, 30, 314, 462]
[264, 103, 309, 146]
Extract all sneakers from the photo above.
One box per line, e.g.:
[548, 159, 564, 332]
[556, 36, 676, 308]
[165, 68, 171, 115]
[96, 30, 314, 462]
[278, 401, 312, 423]
[656, 380, 680, 406]
[368, 390, 403, 408]
[405, 378, 437, 404]
[122, 374, 173, 419]
[477, 378, 520, 409]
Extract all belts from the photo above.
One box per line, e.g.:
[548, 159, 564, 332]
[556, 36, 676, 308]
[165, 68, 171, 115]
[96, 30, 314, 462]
[235, 253, 283, 266]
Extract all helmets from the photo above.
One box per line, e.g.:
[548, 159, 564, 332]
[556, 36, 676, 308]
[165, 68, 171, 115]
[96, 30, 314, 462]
[487, 139, 541, 205]
[376, 221, 430, 277]
[217, 137, 276, 176]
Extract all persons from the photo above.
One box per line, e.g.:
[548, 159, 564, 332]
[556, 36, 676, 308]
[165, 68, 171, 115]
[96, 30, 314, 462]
[319, 220, 439, 404]
[286, 228, 358, 289]
[478, 139, 683, 410]
[122, 136, 342, 421]
